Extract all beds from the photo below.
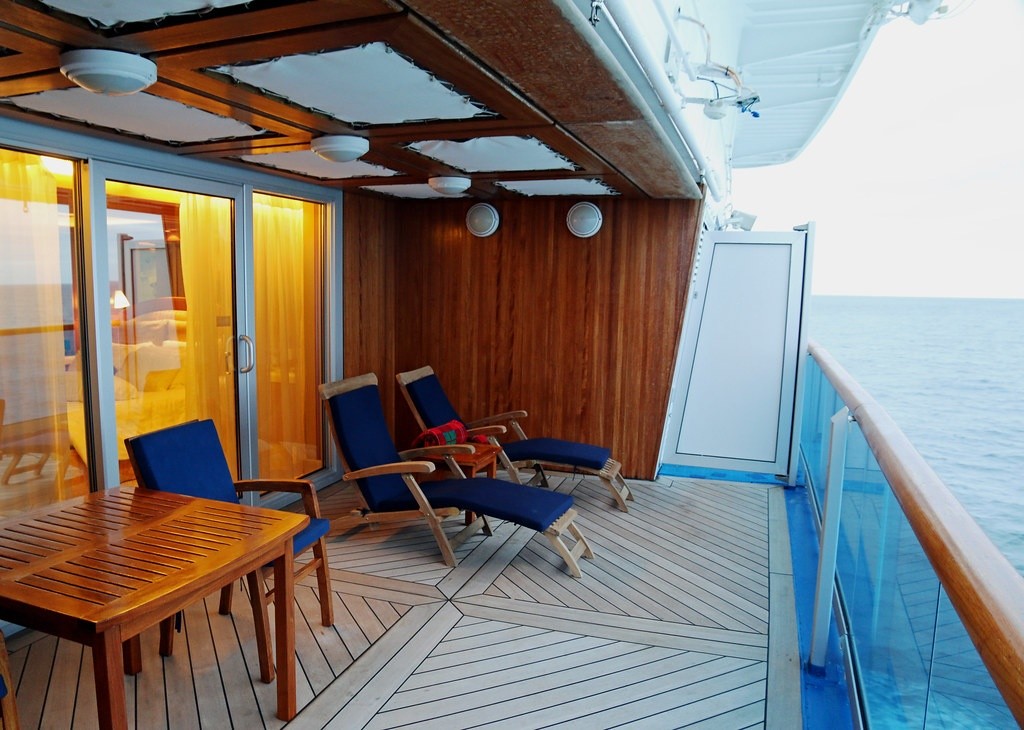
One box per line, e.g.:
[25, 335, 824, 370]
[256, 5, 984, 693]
[57, 296, 190, 498]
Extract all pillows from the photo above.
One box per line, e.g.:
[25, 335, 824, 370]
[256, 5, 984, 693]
[119, 346, 185, 390]
[68, 337, 148, 377]
[64, 370, 138, 401]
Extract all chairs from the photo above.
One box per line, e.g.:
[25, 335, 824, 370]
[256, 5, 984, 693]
[396, 365, 634, 514]
[124, 417, 333, 684]
[319, 374, 594, 579]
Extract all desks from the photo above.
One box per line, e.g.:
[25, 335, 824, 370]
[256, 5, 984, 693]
[411, 442, 502, 526]
[0, 484, 315, 730]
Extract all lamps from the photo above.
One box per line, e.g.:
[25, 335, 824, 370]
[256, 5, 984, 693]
[113, 286, 131, 311]
[310, 137, 370, 164]
[565, 201, 604, 239]
[427, 176, 472, 194]
[59, 47, 157, 95]
[464, 202, 500, 237]
